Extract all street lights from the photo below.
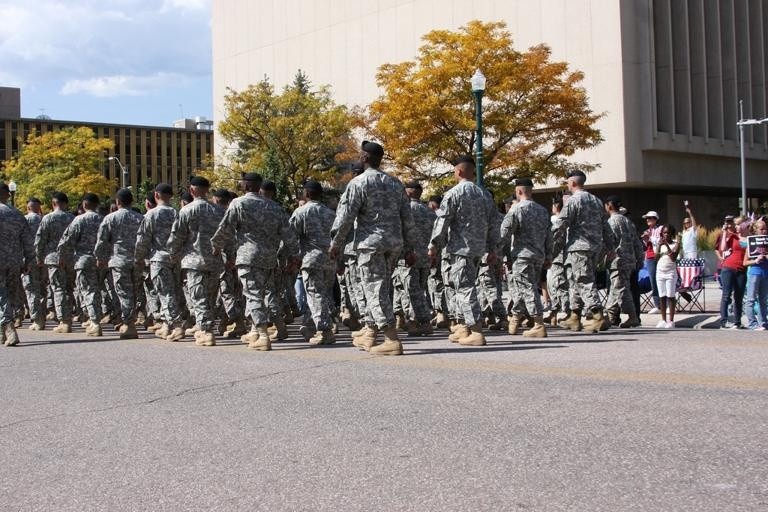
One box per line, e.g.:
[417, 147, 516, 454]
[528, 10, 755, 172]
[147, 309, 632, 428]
[7, 180, 17, 207]
[467, 65, 486, 190]
[107, 155, 128, 188]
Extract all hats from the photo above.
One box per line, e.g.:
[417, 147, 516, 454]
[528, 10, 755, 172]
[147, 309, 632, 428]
[429, 195, 442, 203]
[642, 211, 659, 220]
[0, 182, 9, 191]
[618, 206, 632, 215]
[502, 194, 516, 204]
[552, 170, 586, 204]
[351, 163, 364, 172]
[515, 179, 533, 187]
[302, 179, 323, 194]
[260, 180, 276, 192]
[451, 156, 475, 167]
[361, 141, 384, 155]
[405, 181, 422, 189]
[241, 171, 264, 182]
[26, 175, 238, 212]
[607, 195, 621, 202]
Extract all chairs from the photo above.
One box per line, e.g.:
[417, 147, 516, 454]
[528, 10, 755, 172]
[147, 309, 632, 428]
[676, 256, 706, 313]
[634, 259, 653, 314]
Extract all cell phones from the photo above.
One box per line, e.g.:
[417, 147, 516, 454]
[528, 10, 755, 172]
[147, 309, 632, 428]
[660, 232, 664, 239]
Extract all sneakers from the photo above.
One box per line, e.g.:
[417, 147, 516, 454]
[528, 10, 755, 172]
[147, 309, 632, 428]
[748, 324, 766, 331]
[663, 321, 676, 329]
[648, 307, 662, 314]
[655, 320, 667, 328]
[720, 321, 738, 330]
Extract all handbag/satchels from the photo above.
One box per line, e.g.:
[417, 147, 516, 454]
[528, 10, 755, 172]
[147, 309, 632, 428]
[647, 241, 656, 259]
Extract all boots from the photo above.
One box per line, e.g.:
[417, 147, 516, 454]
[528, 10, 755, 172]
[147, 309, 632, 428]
[430, 309, 620, 335]
[370, 328, 403, 355]
[353, 326, 377, 353]
[0, 306, 216, 346]
[299, 307, 433, 345]
[522, 315, 547, 337]
[218, 304, 302, 350]
[449, 320, 486, 346]
[620, 312, 640, 328]
